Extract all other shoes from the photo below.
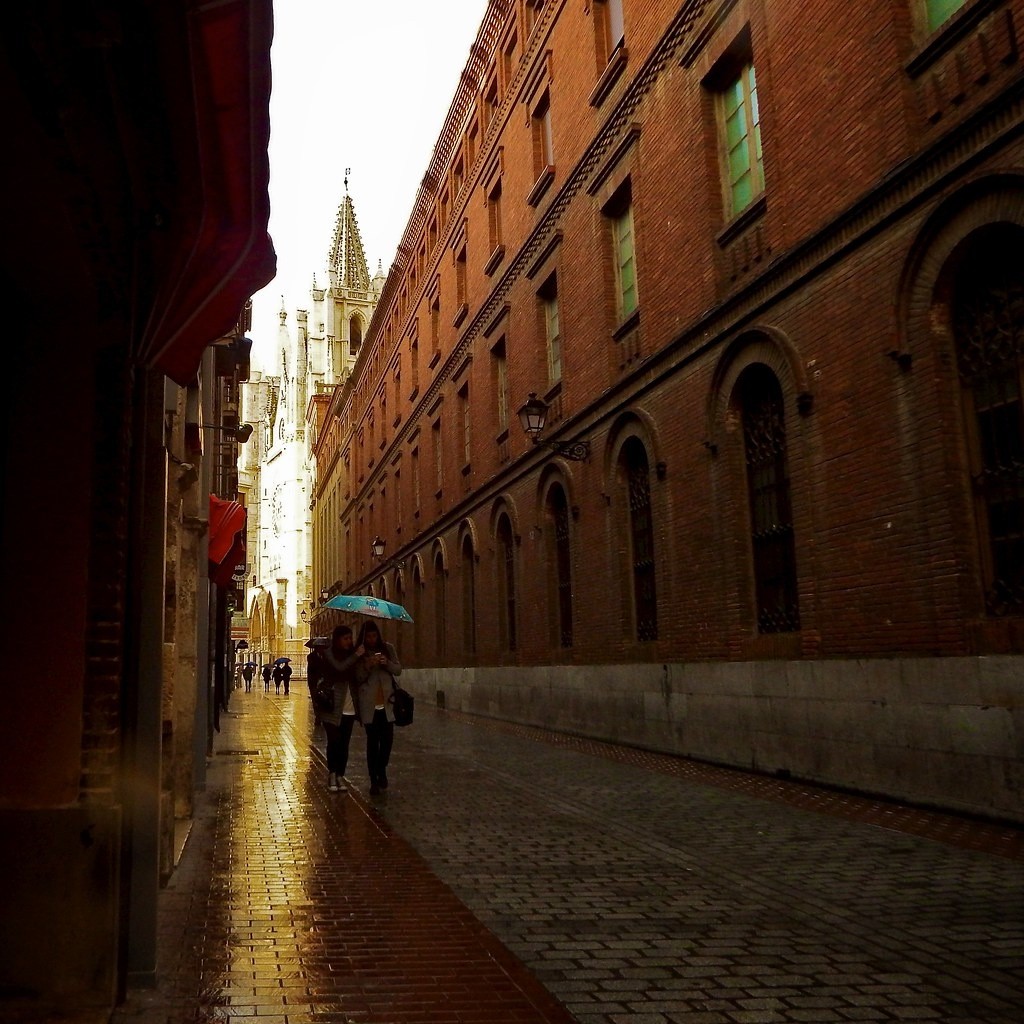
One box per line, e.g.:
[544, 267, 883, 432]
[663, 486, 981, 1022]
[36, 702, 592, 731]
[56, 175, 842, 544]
[370, 782, 388, 793]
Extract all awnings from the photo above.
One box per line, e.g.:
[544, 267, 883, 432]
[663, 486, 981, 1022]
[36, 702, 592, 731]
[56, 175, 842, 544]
[206, 493, 248, 590]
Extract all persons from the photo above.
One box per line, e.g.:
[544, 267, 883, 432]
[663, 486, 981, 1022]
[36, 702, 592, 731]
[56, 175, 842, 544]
[307, 624, 358, 792]
[262, 667, 271, 692]
[253, 665, 256, 676]
[307, 645, 331, 726]
[244, 664, 252, 692]
[346, 619, 402, 797]
[237, 668, 242, 688]
[272, 664, 282, 695]
[282, 662, 292, 696]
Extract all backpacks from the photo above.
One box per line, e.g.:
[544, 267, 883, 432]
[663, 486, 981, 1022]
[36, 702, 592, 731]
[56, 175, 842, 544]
[393, 687, 415, 727]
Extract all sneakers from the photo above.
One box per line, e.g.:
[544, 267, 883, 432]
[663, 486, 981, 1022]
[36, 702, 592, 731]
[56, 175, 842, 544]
[327, 775, 338, 792]
[337, 777, 347, 791]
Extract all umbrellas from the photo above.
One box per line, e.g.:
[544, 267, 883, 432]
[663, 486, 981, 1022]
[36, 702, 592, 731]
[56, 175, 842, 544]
[262, 664, 274, 668]
[245, 662, 258, 666]
[235, 661, 244, 668]
[273, 658, 292, 664]
[321, 593, 415, 646]
[304, 636, 332, 649]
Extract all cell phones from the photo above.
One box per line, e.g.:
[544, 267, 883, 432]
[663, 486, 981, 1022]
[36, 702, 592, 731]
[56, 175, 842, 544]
[374, 653, 381, 655]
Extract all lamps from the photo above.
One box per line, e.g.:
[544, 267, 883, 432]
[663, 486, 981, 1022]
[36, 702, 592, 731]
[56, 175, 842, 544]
[516, 391, 592, 466]
[202, 424, 254, 442]
[372, 535, 406, 570]
[301, 609, 308, 623]
[227, 603, 235, 612]
[528, 524, 542, 540]
[321, 587, 329, 603]
